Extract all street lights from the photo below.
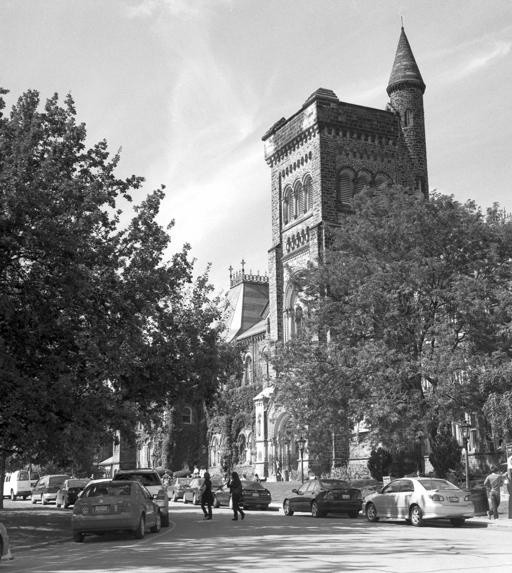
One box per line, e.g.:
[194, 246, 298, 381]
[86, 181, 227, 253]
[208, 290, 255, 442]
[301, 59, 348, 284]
[296, 436, 307, 486]
[459, 420, 471, 492]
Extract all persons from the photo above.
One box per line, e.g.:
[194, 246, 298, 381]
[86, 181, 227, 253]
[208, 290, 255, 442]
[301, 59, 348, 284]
[162, 470, 172, 501]
[227, 471, 246, 521]
[242, 473, 249, 480]
[483, 463, 505, 519]
[253, 473, 261, 484]
[199, 472, 215, 519]
[506, 468, 511, 519]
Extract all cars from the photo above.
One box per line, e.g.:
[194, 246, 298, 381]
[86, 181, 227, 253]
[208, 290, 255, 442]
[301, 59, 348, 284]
[358, 475, 475, 527]
[3, 468, 169, 543]
[282, 478, 363, 520]
[169, 474, 272, 510]
